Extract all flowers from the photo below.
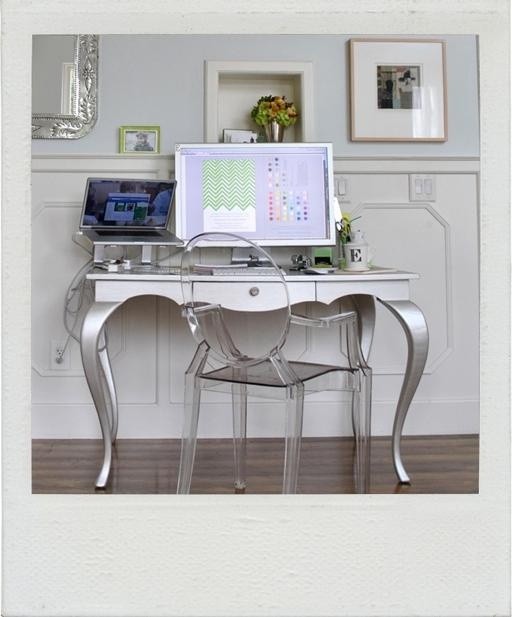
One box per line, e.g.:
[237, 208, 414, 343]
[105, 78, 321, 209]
[251, 95, 298, 126]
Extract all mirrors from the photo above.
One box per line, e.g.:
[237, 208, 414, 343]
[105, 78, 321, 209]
[32, 34, 98, 139]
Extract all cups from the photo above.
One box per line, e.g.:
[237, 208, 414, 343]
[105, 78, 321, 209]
[344, 242, 376, 272]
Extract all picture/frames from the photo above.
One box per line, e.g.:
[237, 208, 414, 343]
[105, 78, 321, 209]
[221, 128, 251, 143]
[348, 38, 449, 142]
[119, 125, 160, 155]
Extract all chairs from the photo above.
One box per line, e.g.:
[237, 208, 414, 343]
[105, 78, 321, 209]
[176, 232, 373, 494]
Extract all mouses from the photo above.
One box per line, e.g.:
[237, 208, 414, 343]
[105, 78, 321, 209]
[304, 266, 329, 274]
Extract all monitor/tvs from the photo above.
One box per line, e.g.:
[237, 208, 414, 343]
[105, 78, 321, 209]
[175, 142, 337, 265]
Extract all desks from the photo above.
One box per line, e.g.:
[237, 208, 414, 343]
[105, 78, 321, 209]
[80, 265, 429, 488]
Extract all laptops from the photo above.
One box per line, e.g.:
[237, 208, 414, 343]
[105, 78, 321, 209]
[79, 176, 184, 243]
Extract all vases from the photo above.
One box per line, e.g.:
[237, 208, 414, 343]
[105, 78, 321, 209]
[264, 121, 284, 142]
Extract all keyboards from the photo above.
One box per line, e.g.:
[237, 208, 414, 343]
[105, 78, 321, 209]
[212, 266, 287, 275]
